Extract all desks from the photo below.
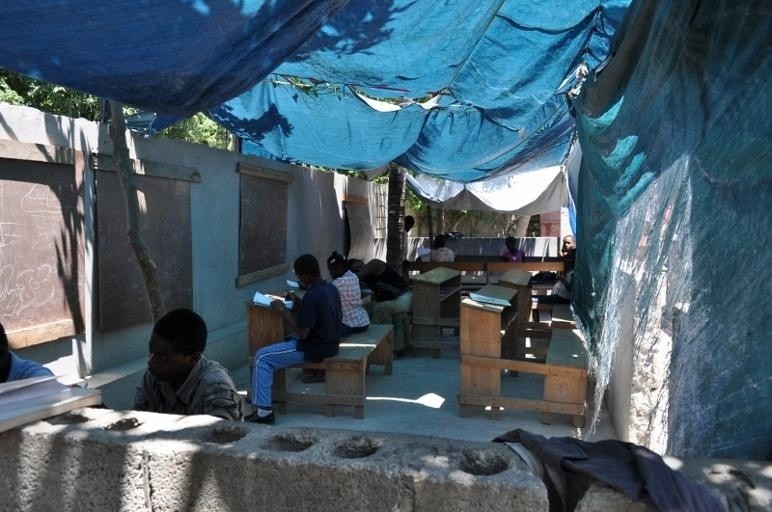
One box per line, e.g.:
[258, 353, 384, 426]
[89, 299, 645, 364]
[247, 288, 301, 415]
[412, 266, 461, 358]
[498, 268, 532, 358]
[457, 284, 519, 418]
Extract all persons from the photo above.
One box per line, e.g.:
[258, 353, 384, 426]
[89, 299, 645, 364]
[136, 309, 244, 421]
[419, 234, 455, 262]
[243, 254, 344, 423]
[403, 216, 415, 264]
[1, 323, 54, 382]
[349, 258, 412, 323]
[502, 237, 525, 261]
[329, 250, 371, 333]
[540, 255, 576, 302]
[562, 236, 576, 254]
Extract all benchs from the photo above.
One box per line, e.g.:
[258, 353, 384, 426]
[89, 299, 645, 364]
[542, 326, 589, 427]
[322, 324, 394, 419]
[553, 305, 577, 327]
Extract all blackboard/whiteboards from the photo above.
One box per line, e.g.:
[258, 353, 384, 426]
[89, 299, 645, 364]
[235, 161, 294, 287]
[92, 153, 201, 334]
[0, 140, 85, 350]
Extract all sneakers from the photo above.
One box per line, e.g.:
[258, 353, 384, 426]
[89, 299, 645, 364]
[244, 410, 276, 422]
